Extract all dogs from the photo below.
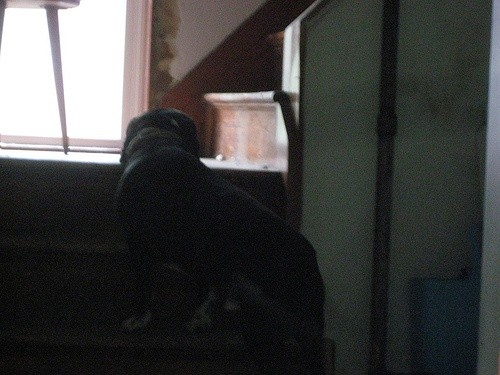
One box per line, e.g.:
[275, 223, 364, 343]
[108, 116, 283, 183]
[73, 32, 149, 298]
[114, 108, 325, 375]
